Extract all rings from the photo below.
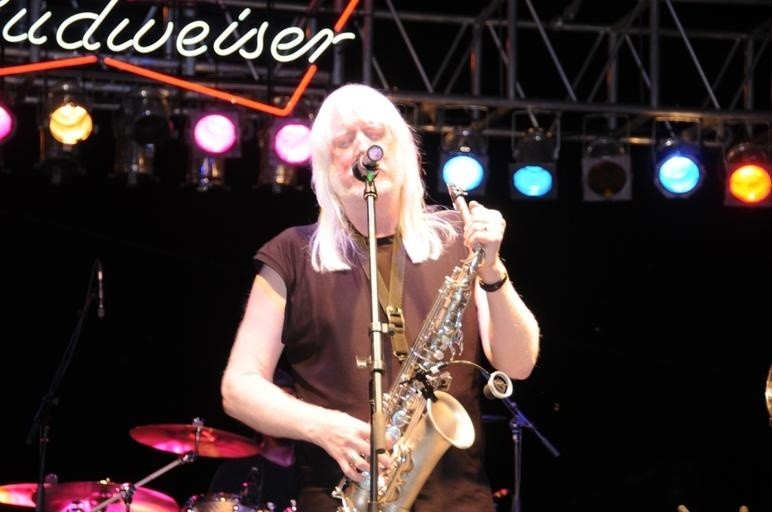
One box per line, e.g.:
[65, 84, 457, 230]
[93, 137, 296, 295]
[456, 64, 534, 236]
[484, 224, 487, 231]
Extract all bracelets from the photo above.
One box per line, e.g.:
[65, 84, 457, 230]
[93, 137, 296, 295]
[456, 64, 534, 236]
[478, 273, 507, 292]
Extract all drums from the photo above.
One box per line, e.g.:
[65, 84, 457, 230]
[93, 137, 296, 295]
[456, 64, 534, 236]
[182, 493, 298, 510]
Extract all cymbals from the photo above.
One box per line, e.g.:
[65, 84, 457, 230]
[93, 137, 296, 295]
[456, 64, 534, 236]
[0, 481, 59, 508]
[132, 425, 261, 458]
[33, 482, 181, 511]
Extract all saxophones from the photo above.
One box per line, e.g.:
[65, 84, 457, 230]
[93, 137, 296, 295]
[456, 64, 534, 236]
[333, 183, 486, 511]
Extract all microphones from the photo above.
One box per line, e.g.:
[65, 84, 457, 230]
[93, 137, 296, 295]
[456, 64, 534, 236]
[98, 262, 105, 318]
[352, 145, 383, 181]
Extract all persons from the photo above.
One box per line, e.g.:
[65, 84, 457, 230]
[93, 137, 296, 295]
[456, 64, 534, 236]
[208, 380, 303, 511]
[219, 83, 540, 512]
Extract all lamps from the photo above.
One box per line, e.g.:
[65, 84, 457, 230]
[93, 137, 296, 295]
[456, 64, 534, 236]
[1, 70, 771, 212]
